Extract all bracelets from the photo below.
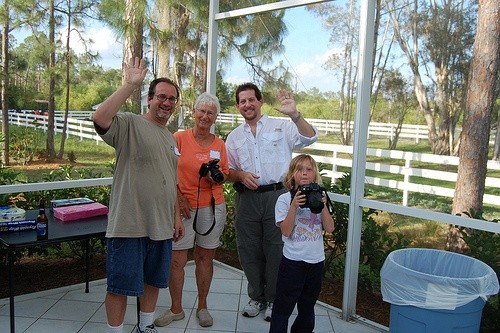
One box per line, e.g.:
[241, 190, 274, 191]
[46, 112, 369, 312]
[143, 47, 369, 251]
[291, 112, 301, 123]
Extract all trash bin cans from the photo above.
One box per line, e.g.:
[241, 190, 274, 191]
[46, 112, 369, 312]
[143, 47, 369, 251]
[389, 248, 493, 333]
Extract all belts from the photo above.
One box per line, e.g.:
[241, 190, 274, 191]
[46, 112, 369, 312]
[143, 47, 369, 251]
[243, 182, 284, 193]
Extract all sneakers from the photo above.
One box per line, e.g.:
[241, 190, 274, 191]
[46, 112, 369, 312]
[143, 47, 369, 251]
[136, 323, 159, 333]
[263, 302, 273, 320]
[154, 310, 185, 326]
[241, 299, 267, 317]
[195, 308, 213, 327]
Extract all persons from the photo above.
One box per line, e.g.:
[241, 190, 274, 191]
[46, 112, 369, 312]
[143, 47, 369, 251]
[153, 92, 229, 327]
[224, 82, 319, 321]
[93, 57, 181, 333]
[268, 153, 334, 333]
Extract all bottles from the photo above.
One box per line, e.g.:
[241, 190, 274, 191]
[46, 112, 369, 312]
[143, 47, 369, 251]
[36, 199, 48, 240]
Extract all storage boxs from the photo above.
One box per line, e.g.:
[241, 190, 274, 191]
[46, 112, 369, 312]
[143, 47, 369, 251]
[0, 196, 108, 231]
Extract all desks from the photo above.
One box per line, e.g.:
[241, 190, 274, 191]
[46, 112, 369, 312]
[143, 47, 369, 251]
[0, 208, 109, 333]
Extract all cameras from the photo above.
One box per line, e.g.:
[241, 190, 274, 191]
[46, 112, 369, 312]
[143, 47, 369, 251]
[199, 160, 225, 184]
[298, 182, 324, 213]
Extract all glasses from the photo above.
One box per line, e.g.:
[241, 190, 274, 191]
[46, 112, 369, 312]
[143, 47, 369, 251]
[154, 94, 178, 104]
